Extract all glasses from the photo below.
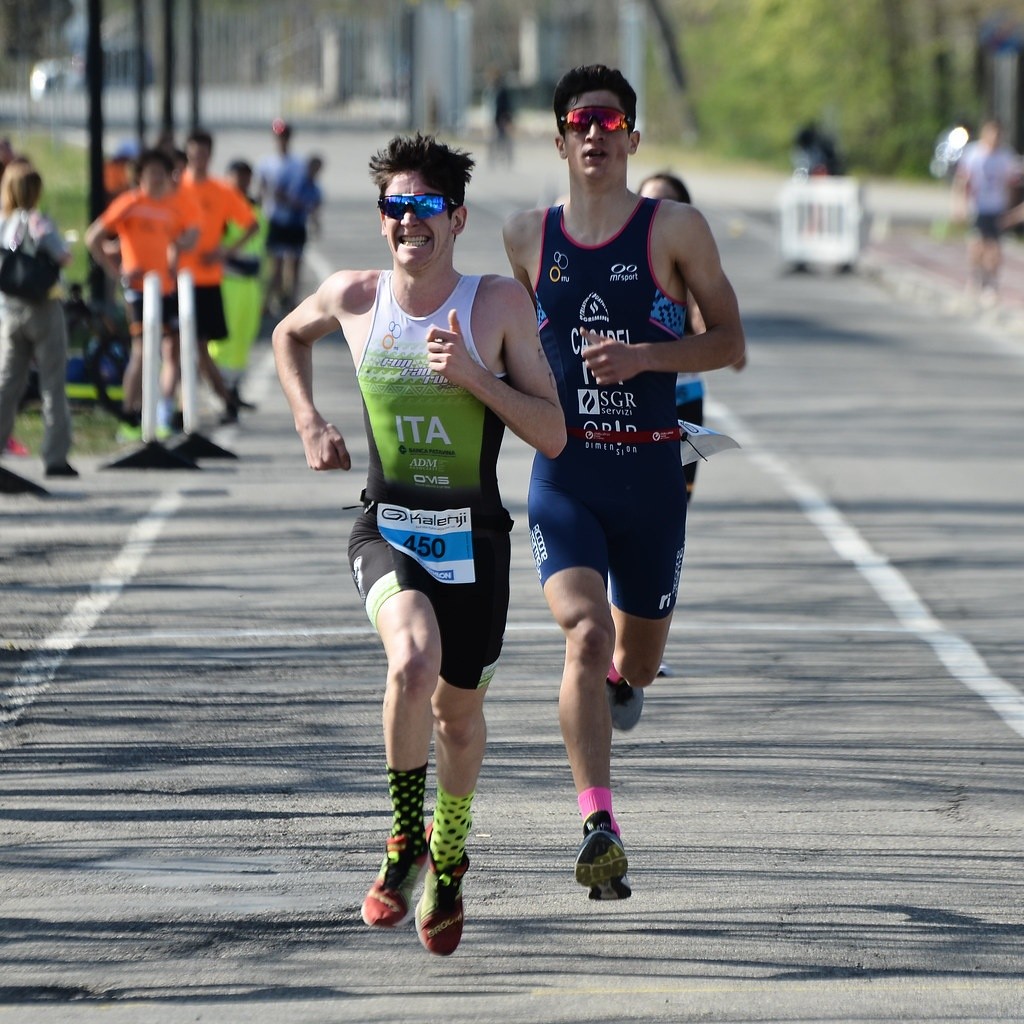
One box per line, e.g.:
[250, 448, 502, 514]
[557, 105, 635, 135]
[377, 192, 455, 220]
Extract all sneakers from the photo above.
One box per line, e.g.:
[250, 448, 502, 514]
[606, 660, 644, 730]
[361, 834, 429, 928]
[414, 818, 470, 957]
[574, 809, 632, 900]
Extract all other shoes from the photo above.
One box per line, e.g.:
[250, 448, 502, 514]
[46, 463, 78, 477]
[656, 662, 671, 676]
[154, 426, 168, 441]
[114, 420, 142, 444]
[220, 387, 239, 422]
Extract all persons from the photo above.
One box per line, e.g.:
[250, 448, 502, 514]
[503, 64, 746, 901]
[272, 130, 567, 956]
[0, 135, 80, 479]
[84, 126, 271, 443]
[483, 66, 516, 169]
[638, 174, 746, 502]
[951, 117, 1024, 301]
[791, 121, 840, 178]
[258, 122, 325, 315]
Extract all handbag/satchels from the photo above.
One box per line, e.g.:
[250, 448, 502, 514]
[0, 209, 59, 300]
